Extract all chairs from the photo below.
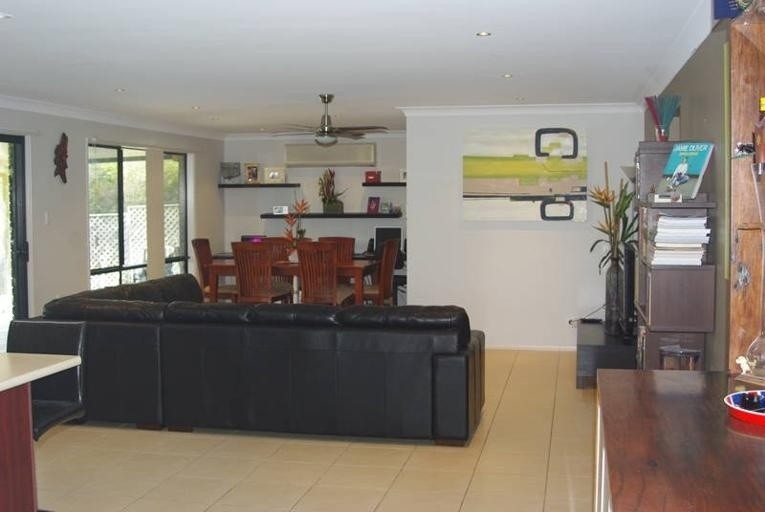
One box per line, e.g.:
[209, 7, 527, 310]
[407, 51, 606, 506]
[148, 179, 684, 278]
[6, 319, 87, 441]
[189, 234, 400, 309]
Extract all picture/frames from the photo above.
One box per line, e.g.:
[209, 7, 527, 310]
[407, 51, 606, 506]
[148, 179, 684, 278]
[243, 161, 264, 186]
[399, 169, 406, 183]
[263, 165, 286, 184]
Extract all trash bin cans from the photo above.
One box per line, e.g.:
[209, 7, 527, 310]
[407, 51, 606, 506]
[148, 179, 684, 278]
[659, 346, 702, 370]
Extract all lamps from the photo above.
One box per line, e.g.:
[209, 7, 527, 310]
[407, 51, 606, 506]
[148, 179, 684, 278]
[316, 131, 338, 147]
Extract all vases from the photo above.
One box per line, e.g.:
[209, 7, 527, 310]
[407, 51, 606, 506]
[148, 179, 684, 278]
[601, 255, 626, 339]
[323, 200, 344, 214]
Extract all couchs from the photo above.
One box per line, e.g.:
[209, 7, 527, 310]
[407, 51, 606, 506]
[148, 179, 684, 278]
[42, 271, 491, 445]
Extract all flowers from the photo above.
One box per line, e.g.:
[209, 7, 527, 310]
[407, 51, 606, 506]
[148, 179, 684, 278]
[578, 169, 638, 269]
[315, 169, 344, 201]
[291, 199, 307, 231]
[280, 215, 297, 250]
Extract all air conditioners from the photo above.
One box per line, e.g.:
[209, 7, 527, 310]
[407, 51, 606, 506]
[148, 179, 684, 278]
[281, 142, 377, 169]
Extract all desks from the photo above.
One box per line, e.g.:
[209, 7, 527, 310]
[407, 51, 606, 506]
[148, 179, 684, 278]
[593, 367, 765, 511]
[0, 345, 84, 510]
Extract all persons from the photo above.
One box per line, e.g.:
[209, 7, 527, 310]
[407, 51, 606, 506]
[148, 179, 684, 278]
[667, 155, 689, 190]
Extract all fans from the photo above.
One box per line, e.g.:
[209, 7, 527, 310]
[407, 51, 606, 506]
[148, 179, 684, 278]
[269, 93, 392, 142]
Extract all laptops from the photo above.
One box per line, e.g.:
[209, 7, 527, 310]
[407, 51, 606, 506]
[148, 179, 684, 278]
[366, 226, 403, 256]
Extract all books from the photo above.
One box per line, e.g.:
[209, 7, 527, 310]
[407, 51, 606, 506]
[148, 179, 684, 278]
[640, 207, 711, 267]
[654, 141, 714, 200]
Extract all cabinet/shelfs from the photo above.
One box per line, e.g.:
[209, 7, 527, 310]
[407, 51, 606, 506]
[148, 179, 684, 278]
[622, 142, 720, 368]
[576, 318, 639, 391]
